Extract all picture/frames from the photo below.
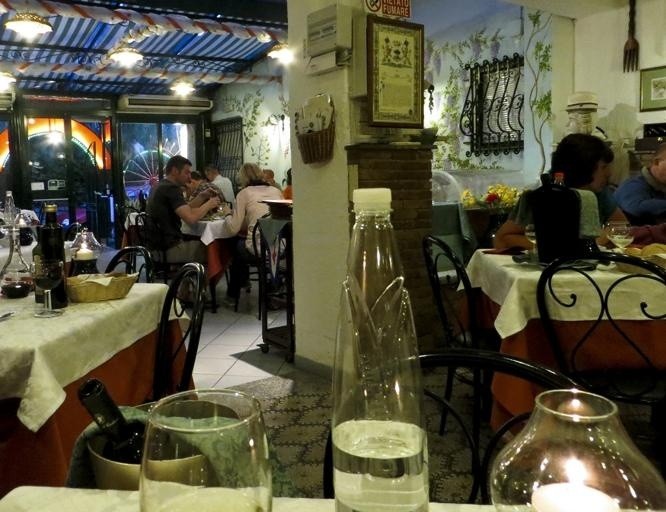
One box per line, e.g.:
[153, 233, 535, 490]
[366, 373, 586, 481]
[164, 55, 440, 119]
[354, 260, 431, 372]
[366, 13, 425, 131]
[638, 65, 666, 112]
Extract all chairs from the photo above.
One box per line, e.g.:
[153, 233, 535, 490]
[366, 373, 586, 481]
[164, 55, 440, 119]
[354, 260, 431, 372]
[152, 262, 208, 401]
[419, 233, 503, 436]
[117, 205, 138, 274]
[105, 246, 153, 282]
[535, 251, 666, 439]
[234, 208, 270, 321]
[322, 350, 579, 504]
[135, 212, 183, 282]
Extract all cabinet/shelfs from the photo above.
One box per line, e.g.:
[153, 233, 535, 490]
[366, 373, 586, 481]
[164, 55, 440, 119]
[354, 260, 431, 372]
[257, 217, 295, 363]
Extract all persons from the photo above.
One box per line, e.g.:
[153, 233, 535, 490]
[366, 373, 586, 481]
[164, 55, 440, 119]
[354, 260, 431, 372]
[204, 163, 235, 203]
[184, 170, 225, 214]
[144, 155, 207, 309]
[262, 169, 283, 192]
[491, 133, 632, 253]
[222, 162, 286, 306]
[612, 141, 666, 227]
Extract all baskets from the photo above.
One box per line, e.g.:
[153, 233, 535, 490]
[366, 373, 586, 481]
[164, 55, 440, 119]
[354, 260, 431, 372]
[65, 271, 140, 304]
[295, 93, 335, 165]
[610, 243, 666, 275]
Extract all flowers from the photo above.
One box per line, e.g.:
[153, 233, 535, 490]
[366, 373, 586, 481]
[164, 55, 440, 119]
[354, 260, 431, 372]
[462, 182, 519, 211]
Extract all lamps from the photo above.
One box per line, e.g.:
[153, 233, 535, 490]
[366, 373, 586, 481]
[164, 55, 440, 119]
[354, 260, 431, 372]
[5, 12, 53, 35]
[107, 45, 143, 65]
[265, 43, 288, 59]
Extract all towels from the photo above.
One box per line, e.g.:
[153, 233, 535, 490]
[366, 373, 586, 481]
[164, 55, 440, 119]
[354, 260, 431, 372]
[571, 186, 602, 240]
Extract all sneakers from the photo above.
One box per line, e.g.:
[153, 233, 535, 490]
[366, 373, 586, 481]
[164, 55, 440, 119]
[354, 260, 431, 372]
[223, 295, 236, 306]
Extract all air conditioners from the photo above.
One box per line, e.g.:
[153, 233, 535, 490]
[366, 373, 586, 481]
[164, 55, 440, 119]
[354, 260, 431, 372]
[0, 90, 16, 110]
[117, 93, 213, 115]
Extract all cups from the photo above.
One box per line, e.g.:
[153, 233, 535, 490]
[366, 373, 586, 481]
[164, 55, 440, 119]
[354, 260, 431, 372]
[607, 222, 634, 254]
[525, 224, 537, 258]
[30, 260, 64, 318]
[219, 203, 227, 211]
[140, 389, 272, 511]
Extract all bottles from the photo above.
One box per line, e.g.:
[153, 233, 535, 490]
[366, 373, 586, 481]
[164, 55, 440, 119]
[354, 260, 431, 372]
[41, 204, 68, 310]
[331, 188, 431, 512]
[76, 378, 145, 465]
[3, 190, 16, 224]
[32, 225, 46, 304]
